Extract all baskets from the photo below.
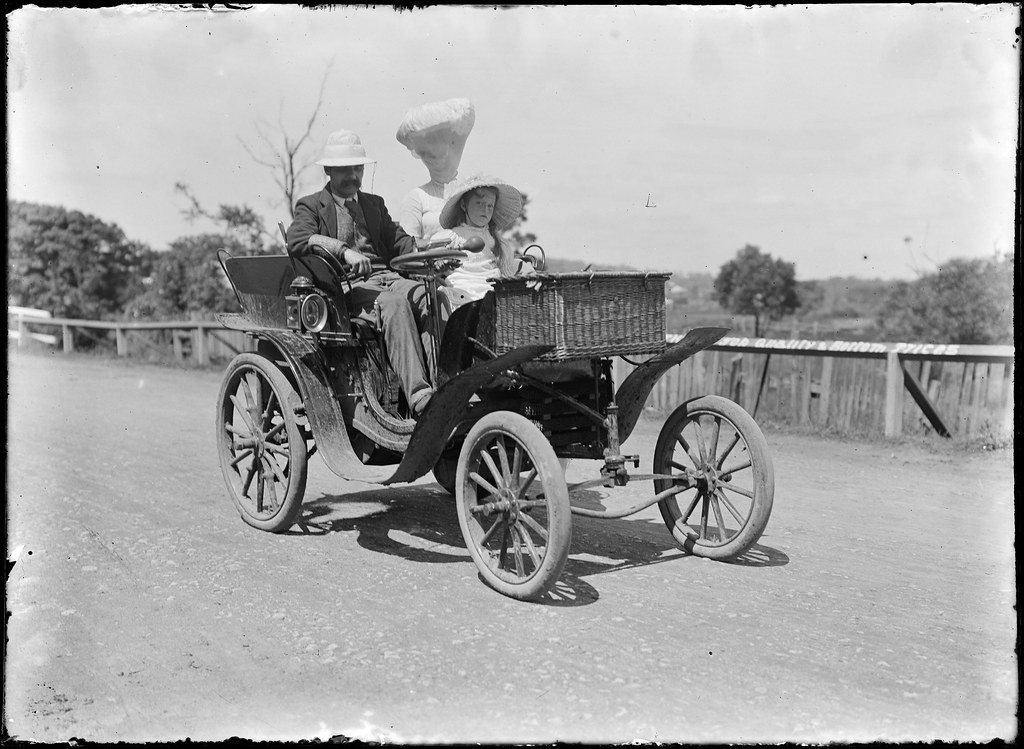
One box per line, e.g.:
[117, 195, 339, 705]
[489, 270, 672, 357]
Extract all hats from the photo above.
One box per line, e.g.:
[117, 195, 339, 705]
[316, 130, 378, 167]
[438, 180, 522, 229]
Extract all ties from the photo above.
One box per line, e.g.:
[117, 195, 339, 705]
[343, 200, 372, 242]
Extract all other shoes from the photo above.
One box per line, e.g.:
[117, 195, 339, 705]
[413, 393, 433, 416]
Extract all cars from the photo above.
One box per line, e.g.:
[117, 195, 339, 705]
[216, 222, 774, 603]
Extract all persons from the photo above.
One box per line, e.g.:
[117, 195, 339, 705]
[288, 130, 451, 417]
[429, 178, 542, 316]
[395, 98, 535, 273]
